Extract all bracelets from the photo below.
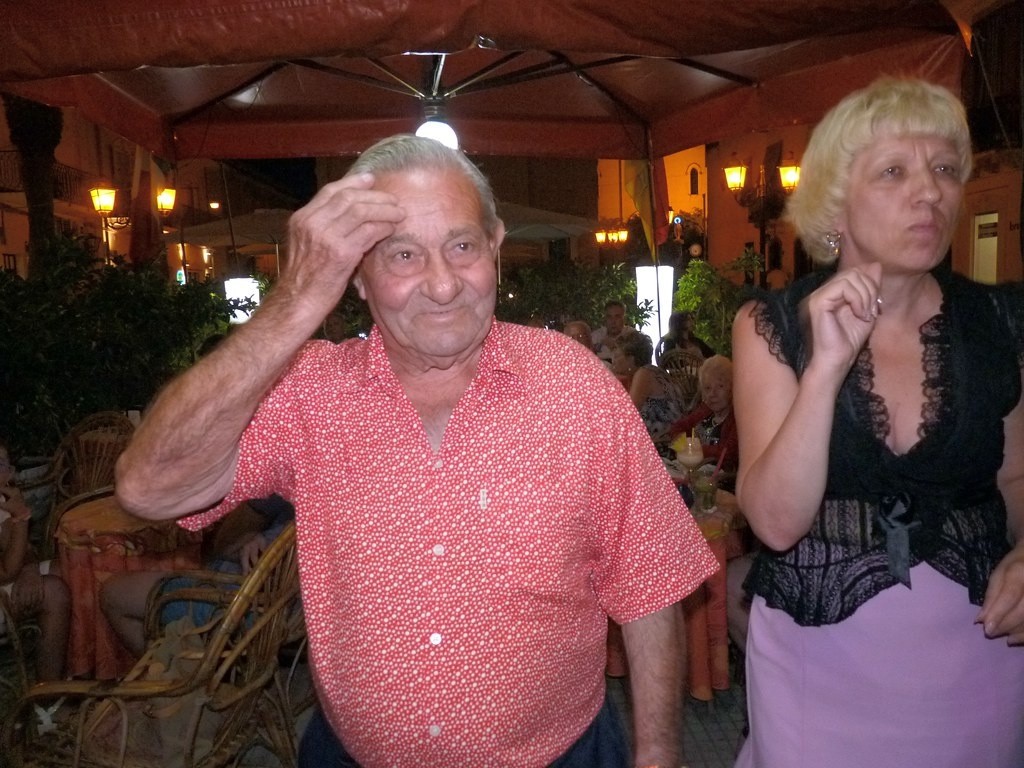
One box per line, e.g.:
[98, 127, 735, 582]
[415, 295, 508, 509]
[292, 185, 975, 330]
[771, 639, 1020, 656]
[12, 508, 32, 523]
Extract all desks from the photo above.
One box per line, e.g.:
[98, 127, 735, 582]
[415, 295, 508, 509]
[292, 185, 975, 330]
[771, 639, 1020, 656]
[605, 488, 741, 700]
[55, 496, 262, 681]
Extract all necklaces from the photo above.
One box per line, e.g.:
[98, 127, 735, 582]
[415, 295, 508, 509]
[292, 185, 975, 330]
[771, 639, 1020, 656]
[712, 414, 727, 428]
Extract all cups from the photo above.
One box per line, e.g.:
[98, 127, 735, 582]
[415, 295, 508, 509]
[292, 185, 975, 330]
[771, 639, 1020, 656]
[689, 475, 717, 514]
[128, 410, 140, 424]
[677, 437, 704, 472]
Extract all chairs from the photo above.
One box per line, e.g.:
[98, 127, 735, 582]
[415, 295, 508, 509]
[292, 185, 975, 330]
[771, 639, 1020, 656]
[0, 519, 304, 768]
[659, 349, 706, 408]
[655, 332, 675, 366]
[5, 410, 136, 563]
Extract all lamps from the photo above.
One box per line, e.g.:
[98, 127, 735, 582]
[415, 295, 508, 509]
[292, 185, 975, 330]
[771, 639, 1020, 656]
[668, 206, 674, 226]
[594, 225, 628, 265]
[88, 179, 130, 230]
[157, 186, 177, 217]
[721, 148, 801, 292]
[415, 101, 458, 150]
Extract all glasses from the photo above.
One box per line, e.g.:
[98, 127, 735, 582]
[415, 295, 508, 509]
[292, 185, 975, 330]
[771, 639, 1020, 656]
[361, 232, 493, 279]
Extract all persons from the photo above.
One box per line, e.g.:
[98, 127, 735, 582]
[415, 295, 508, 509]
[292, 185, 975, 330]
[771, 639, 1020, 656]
[733, 72, 1024, 768]
[0, 441, 82, 738]
[116, 134, 739, 768]
[322, 312, 349, 345]
[99, 493, 295, 704]
